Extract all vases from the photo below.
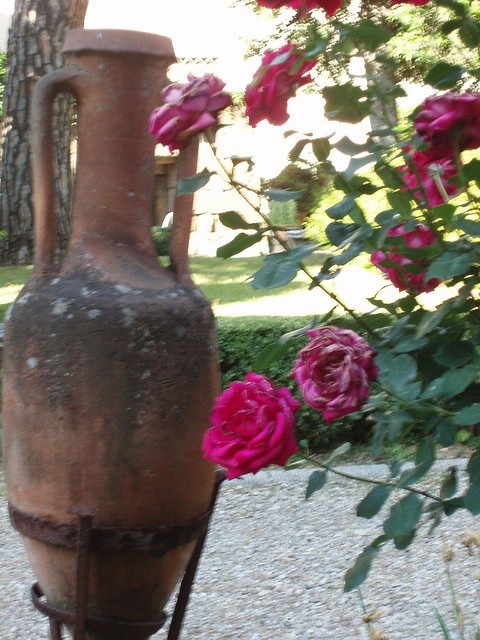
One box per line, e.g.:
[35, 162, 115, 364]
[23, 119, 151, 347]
[0, 26, 219, 640]
